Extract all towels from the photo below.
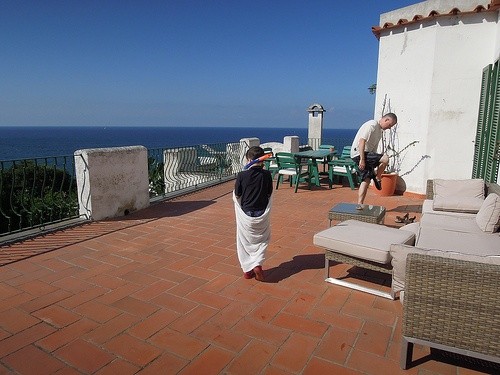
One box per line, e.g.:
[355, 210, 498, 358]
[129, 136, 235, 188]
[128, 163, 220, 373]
[232, 189, 274, 273]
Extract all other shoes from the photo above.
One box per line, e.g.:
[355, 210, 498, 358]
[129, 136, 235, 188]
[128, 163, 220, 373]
[254, 267, 265, 280]
[244, 270, 255, 279]
[373, 177, 382, 190]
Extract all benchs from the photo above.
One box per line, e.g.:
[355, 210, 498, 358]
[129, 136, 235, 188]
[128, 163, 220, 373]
[399, 179, 500, 371]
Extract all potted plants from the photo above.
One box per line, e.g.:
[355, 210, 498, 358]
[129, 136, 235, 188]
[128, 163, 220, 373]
[371, 92, 419, 197]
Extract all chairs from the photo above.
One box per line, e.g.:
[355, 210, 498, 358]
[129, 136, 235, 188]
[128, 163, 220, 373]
[298, 145, 315, 171]
[275, 151, 311, 193]
[262, 147, 283, 183]
[308, 144, 335, 173]
[327, 145, 362, 190]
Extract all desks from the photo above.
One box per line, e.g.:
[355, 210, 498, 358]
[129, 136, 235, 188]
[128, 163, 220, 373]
[289, 149, 339, 188]
[328, 202, 386, 226]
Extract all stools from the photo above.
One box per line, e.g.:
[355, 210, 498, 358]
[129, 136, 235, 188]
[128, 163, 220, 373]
[313, 218, 417, 301]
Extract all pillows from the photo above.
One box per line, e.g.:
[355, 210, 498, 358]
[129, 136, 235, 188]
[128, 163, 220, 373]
[433, 177, 485, 214]
[474, 192, 500, 233]
[387, 241, 500, 293]
[398, 222, 420, 241]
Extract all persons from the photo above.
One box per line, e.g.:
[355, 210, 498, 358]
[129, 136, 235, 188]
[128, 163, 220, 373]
[350, 113, 397, 204]
[233, 146, 273, 280]
